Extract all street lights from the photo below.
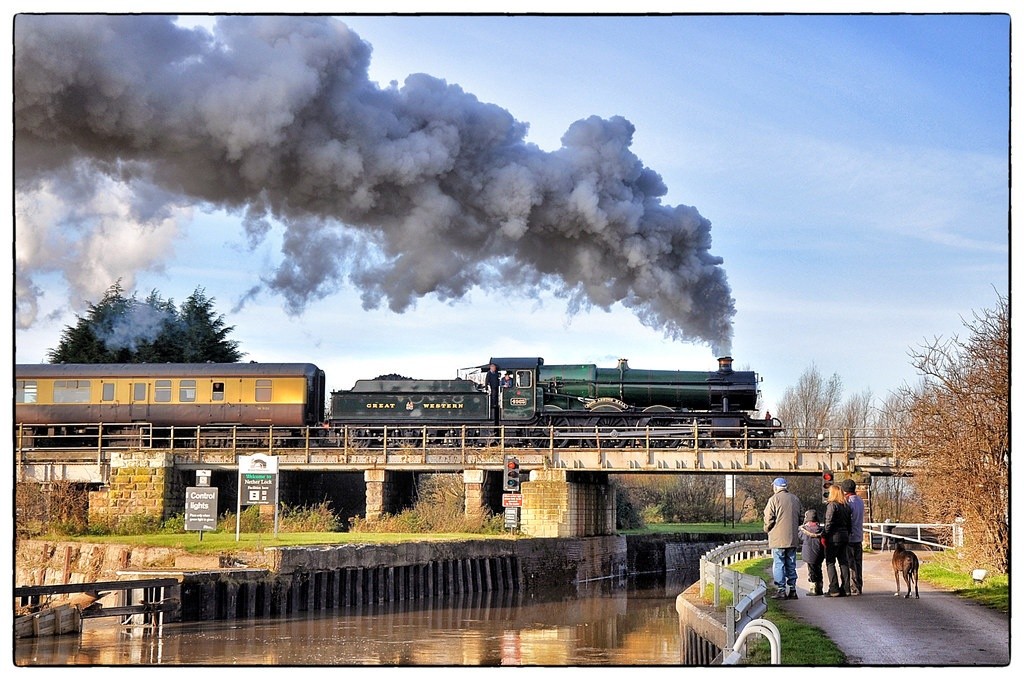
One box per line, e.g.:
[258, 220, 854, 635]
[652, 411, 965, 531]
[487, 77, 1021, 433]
[818, 428, 832, 470]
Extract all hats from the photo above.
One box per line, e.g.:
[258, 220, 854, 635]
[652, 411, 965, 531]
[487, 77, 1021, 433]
[771, 478, 787, 487]
[843, 479, 856, 492]
[804, 510, 819, 523]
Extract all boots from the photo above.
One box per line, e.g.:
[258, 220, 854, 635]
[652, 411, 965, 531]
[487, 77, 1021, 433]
[806, 581, 823, 596]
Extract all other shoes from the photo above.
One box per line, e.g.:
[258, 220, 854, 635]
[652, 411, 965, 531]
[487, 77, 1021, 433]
[838, 590, 851, 597]
[824, 589, 840, 597]
[850, 588, 862, 596]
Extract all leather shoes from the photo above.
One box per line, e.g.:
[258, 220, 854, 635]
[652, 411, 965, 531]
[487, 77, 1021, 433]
[786, 590, 799, 600]
[771, 590, 787, 599]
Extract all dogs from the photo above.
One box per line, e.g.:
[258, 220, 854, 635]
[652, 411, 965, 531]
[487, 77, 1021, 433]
[891, 538, 920, 599]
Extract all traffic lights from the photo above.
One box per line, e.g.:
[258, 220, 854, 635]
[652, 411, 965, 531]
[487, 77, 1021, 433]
[822, 470, 834, 503]
[504, 458, 520, 491]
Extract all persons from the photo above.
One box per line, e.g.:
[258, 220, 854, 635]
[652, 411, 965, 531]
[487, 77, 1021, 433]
[880, 519, 900, 553]
[763, 478, 805, 600]
[485, 363, 513, 409]
[821, 479, 863, 597]
[797, 509, 825, 597]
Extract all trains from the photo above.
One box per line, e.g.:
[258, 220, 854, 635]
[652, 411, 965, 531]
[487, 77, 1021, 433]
[16, 356, 786, 449]
